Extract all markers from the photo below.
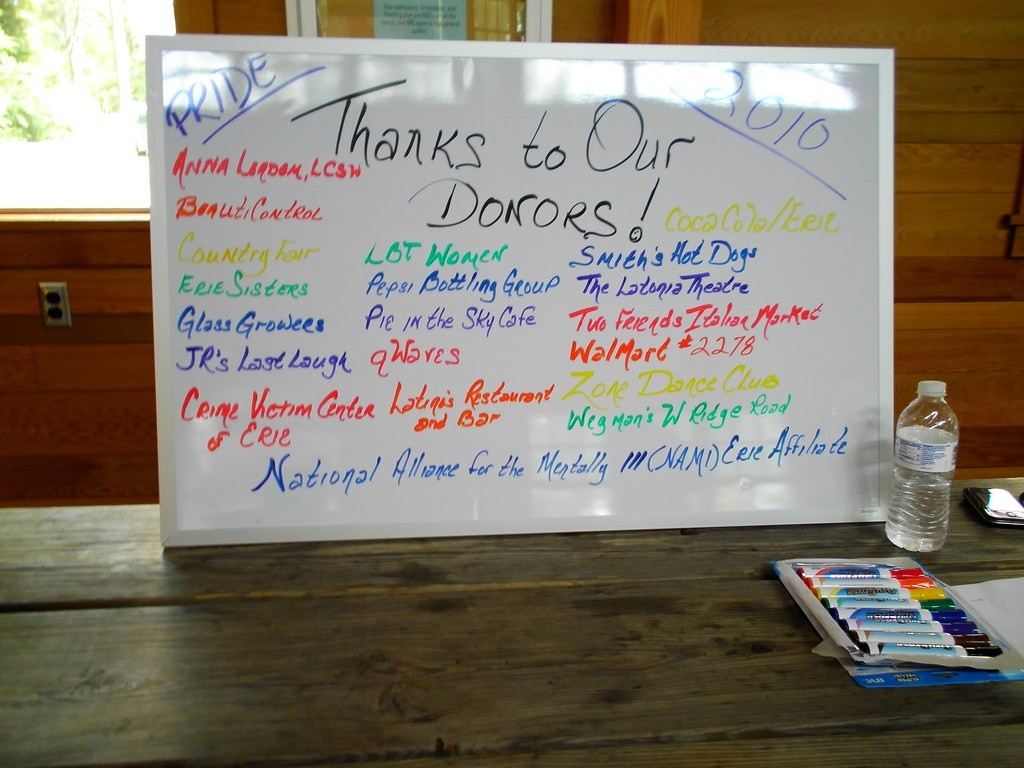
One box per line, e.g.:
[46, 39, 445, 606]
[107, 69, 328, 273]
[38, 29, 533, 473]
[791, 561, 1004, 658]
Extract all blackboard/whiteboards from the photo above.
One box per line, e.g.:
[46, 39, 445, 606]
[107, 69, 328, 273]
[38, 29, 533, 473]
[146, 34, 896, 549]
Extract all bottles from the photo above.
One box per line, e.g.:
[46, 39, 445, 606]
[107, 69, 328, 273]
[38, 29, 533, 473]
[885, 380, 959, 553]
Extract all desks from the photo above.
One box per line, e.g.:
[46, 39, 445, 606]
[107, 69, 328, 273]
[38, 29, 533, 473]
[1, 477, 1023, 768]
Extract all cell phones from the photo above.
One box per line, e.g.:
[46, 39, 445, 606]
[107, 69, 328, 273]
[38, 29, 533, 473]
[962, 487, 1024, 529]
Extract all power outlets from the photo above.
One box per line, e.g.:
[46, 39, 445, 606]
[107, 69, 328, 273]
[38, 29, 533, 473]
[39, 283, 72, 327]
